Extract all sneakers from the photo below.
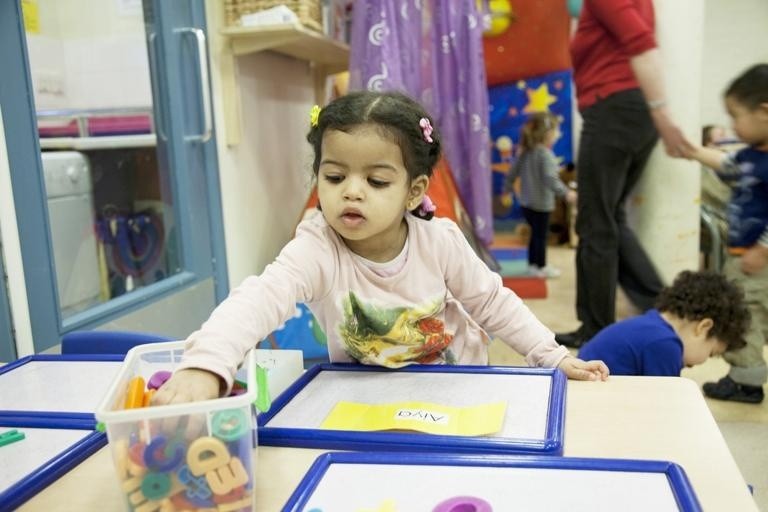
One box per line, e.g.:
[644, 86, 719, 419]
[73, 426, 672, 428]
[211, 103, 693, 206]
[532, 267, 559, 276]
[703, 377, 764, 404]
[554, 324, 602, 349]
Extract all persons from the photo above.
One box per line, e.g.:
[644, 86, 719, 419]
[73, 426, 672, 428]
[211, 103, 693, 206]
[502, 113, 578, 279]
[148, 89, 610, 442]
[682, 61, 768, 405]
[701, 122, 735, 273]
[554, 0, 698, 349]
[577, 269, 752, 378]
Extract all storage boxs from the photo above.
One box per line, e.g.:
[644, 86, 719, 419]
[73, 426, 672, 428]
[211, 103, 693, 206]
[92, 338, 260, 512]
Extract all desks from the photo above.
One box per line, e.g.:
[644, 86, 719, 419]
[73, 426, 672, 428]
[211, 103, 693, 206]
[1, 361, 760, 511]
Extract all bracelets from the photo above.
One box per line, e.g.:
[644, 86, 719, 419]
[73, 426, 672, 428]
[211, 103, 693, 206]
[646, 96, 671, 111]
